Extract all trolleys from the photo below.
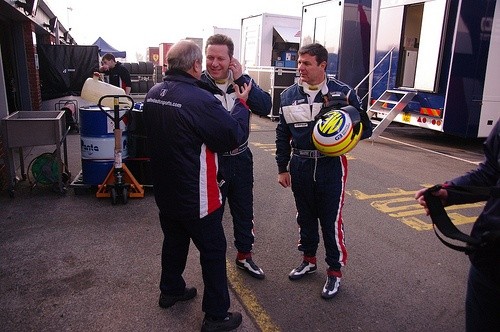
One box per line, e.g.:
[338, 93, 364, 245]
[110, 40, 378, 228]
[68, 94, 154, 205]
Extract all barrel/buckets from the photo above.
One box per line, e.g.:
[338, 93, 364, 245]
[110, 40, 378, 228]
[128, 107, 152, 185]
[130, 79, 154, 93]
[79, 105, 133, 184]
[122, 61, 154, 74]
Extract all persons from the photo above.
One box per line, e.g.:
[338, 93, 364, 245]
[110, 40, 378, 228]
[144, 40, 253, 332]
[198, 34, 272, 279]
[100, 53, 132, 96]
[416, 117, 500, 332]
[274, 44, 373, 299]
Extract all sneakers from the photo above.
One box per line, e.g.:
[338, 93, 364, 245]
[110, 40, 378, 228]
[321, 269, 342, 298]
[288, 257, 317, 280]
[236, 253, 265, 279]
[202, 312, 242, 332]
[159, 287, 197, 308]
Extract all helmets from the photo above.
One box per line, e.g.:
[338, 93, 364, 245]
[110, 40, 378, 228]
[312, 105, 363, 156]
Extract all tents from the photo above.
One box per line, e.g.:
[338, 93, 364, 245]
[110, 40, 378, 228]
[93, 37, 126, 63]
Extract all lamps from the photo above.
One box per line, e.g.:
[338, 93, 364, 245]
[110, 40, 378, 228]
[43, 15, 58, 33]
[14, 0, 40, 18]
[59, 31, 69, 42]
[66, 38, 74, 45]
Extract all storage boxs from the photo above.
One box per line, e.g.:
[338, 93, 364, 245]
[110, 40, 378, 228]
[244, 52, 299, 123]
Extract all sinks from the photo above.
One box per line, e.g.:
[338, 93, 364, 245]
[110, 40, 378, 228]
[2, 111, 67, 148]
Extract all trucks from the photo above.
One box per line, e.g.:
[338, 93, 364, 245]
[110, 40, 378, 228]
[212, 0, 500, 131]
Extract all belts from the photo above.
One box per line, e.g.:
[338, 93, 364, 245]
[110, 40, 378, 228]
[218, 140, 248, 156]
[292, 148, 325, 158]
